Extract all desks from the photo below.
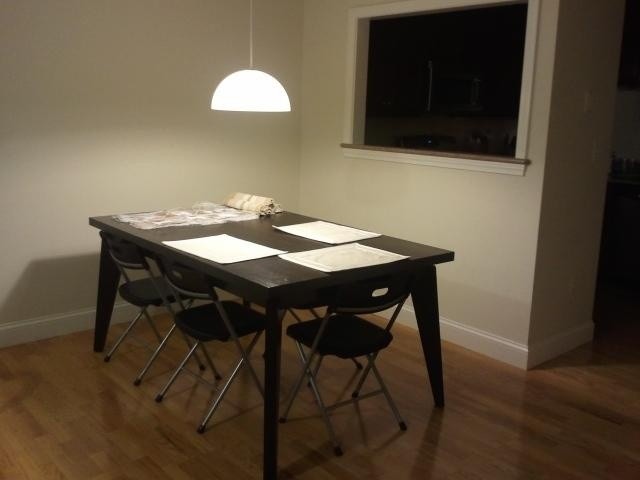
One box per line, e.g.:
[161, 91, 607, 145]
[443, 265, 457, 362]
[87, 199, 453, 480]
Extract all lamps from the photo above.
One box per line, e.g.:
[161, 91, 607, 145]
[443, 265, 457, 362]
[209, 1, 293, 114]
